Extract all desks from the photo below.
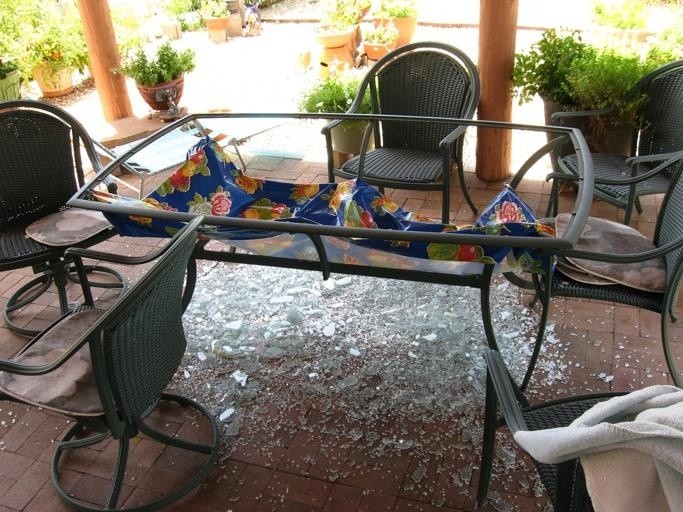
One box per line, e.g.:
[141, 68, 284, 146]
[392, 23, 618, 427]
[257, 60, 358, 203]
[87, 107, 193, 180]
[61, 101, 597, 509]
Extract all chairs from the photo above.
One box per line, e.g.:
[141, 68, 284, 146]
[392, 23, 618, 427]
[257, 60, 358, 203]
[2, 96, 149, 344]
[1, 203, 228, 511]
[464, 338, 683, 511]
[319, 38, 484, 229]
[541, 55, 683, 233]
[500, 145, 683, 399]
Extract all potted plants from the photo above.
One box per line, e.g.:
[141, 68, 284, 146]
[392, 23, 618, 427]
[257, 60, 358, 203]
[108, 45, 200, 112]
[511, 26, 670, 197]
[21, 33, 91, 96]
[1, 50, 22, 104]
[197, 1, 233, 31]
[302, 76, 387, 161]
[305, 0, 423, 74]
